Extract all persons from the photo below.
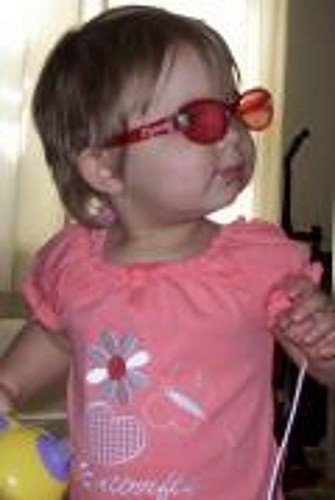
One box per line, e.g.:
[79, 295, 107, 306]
[0, 4, 335, 500]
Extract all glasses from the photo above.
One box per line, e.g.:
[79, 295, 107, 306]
[100, 86, 276, 147]
